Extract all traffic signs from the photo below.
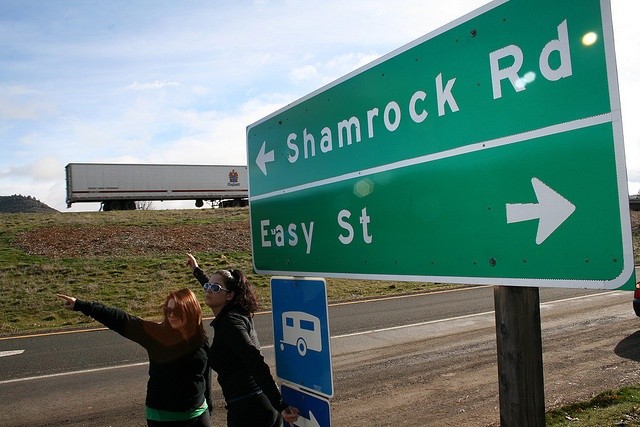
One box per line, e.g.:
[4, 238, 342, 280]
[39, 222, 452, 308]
[244, 0, 636, 291]
[268, 276, 333, 398]
[274, 382, 332, 427]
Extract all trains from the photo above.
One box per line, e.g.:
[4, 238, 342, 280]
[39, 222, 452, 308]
[65, 163, 248, 211]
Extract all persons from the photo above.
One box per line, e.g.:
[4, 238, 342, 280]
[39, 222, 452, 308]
[185, 252, 299, 427]
[56, 288, 213, 427]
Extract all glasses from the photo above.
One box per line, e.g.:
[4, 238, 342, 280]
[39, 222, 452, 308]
[164, 307, 178, 317]
[204, 283, 230, 294]
